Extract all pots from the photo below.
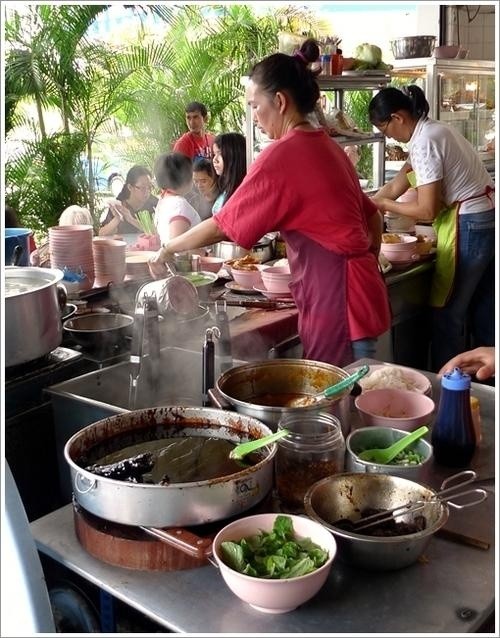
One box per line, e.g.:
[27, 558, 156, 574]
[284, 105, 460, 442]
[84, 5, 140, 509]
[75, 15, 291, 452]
[208, 359, 361, 435]
[122, 302, 210, 334]
[214, 233, 274, 261]
[5, 228, 68, 368]
[64, 405, 278, 526]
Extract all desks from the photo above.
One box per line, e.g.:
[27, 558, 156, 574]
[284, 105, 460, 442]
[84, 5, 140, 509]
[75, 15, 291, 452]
[90, 233, 176, 257]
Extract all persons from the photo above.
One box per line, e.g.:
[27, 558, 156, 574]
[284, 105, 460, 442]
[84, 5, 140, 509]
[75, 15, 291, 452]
[58, 101, 247, 262]
[367, 84, 495, 375]
[343, 144, 364, 180]
[5, 205, 37, 253]
[437, 346, 495, 381]
[146, 38, 393, 371]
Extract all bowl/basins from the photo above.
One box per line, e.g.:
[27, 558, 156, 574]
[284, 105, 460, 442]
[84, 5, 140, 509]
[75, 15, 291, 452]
[178, 271, 218, 300]
[174, 253, 224, 272]
[231, 264, 293, 292]
[213, 512, 337, 614]
[380, 189, 434, 269]
[59, 301, 134, 349]
[355, 388, 435, 431]
[389, 35, 470, 60]
[304, 471, 449, 568]
[47, 225, 158, 292]
[346, 426, 434, 486]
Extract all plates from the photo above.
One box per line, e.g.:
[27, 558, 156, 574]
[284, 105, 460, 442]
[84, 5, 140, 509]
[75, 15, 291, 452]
[225, 281, 293, 300]
[341, 69, 391, 77]
[345, 365, 431, 395]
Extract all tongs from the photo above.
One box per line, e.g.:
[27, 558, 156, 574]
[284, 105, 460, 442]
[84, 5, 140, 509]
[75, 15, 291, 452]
[332, 469, 489, 532]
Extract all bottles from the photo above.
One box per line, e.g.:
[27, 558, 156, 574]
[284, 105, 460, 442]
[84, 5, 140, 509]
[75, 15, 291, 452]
[431, 367, 481, 472]
[317, 44, 344, 76]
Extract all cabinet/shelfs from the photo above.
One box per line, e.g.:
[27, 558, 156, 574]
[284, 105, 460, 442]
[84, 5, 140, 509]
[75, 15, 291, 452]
[376, 58, 495, 172]
[245, 74, 393, 198]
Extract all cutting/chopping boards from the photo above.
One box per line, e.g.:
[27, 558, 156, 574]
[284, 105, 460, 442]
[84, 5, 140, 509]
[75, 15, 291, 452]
[72, 493, 273, 572]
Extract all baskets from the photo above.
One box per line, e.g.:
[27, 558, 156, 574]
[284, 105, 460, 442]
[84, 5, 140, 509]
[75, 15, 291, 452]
[391, 35, 436, 59]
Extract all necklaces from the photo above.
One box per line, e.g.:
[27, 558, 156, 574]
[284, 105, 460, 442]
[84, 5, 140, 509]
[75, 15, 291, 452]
[290, 122, 310, 130]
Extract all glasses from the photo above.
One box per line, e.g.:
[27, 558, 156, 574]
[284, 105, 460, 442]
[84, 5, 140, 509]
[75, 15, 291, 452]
[134, 183, 154, 192]
[378, 115, 392, 138]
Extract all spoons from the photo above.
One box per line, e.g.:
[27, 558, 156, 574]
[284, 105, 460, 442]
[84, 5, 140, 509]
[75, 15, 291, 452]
[358, 426, 429, 464]
